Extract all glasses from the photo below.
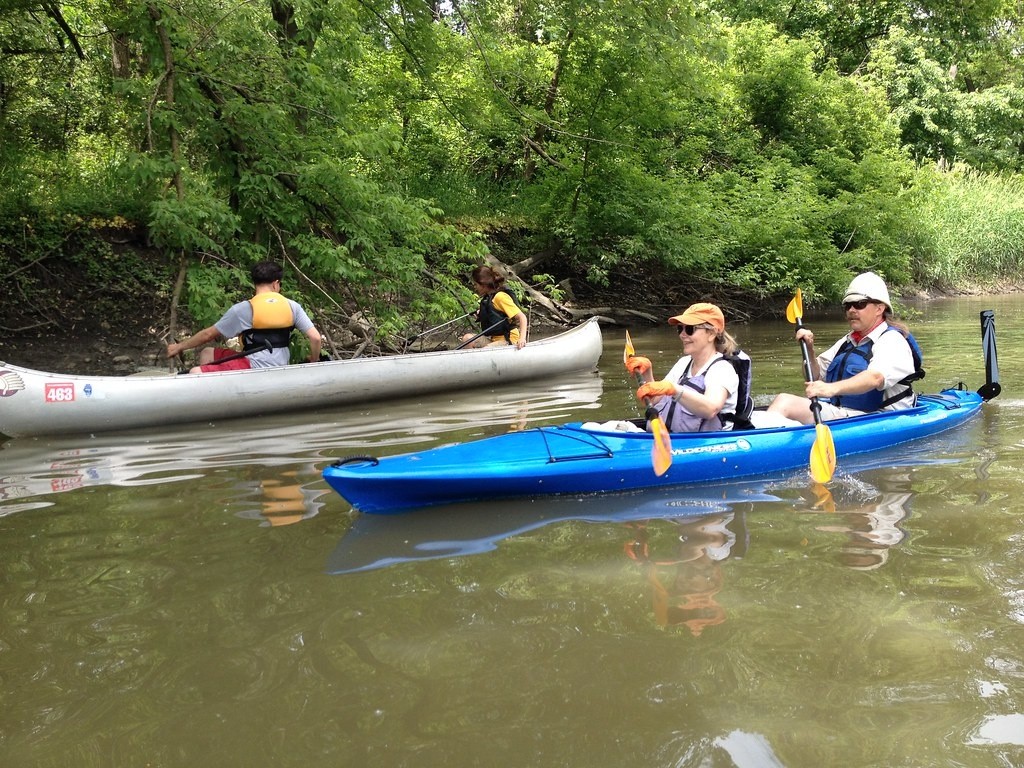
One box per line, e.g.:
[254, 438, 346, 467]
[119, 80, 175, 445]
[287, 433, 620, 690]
[845, 299, 881, 311]
[675, 322, 713, 336]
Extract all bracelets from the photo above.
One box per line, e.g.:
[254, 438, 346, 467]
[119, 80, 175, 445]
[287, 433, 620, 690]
[671, 385, 685, 403]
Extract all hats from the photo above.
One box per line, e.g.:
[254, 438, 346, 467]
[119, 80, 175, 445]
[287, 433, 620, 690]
[668, 303, 725, 334]
[842, 271, 894, 317]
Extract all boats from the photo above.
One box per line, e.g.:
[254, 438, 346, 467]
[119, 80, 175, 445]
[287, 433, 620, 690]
[322, 309, 1002, 515]
[0, 313, 604, 441]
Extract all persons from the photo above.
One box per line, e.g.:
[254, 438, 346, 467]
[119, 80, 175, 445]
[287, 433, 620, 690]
[766, 269, 916, 425]
[626, 302, 740, 433]
[463, 265, 527, 349]
[165, 261, 321, 374]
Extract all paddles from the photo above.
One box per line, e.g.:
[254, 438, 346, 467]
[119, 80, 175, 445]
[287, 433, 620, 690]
[452, 317, 507, 351]
[620, 324, 677, 483]
[402, 310, 477, 346]
[781, 283, 841, 488]
[167, 339, 273, 375]
